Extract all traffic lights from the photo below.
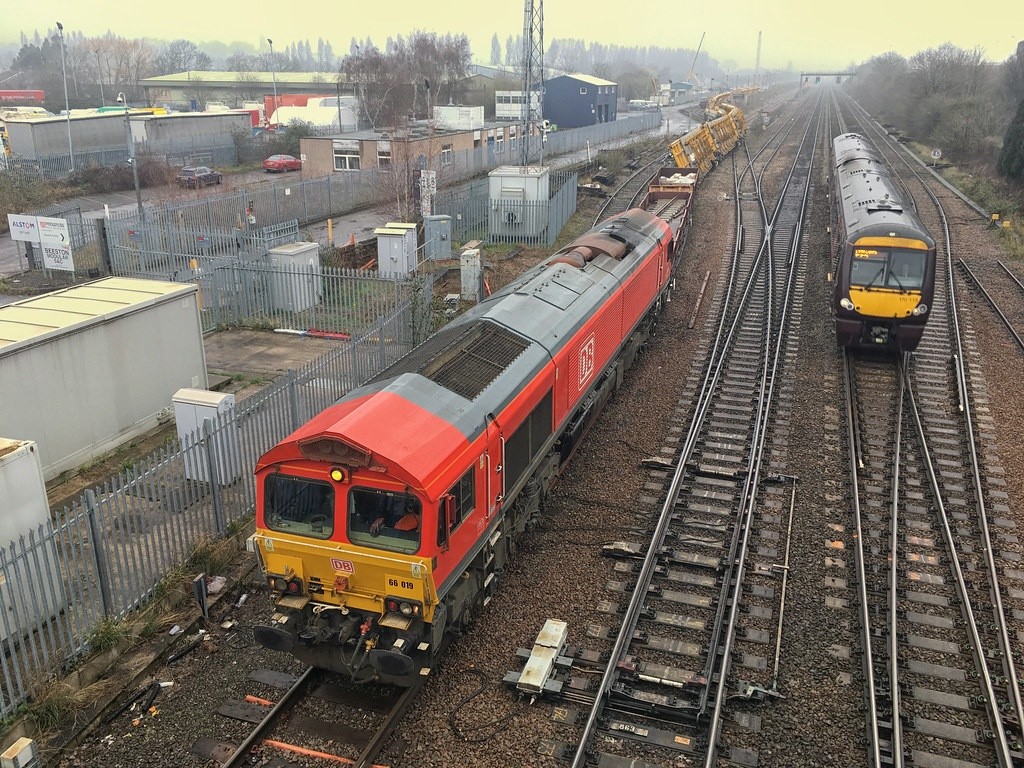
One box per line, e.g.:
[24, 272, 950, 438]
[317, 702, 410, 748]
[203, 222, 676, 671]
[837, 77, 841, 84]
[816, 77, 820, 84]
[805, 77, 808, 84]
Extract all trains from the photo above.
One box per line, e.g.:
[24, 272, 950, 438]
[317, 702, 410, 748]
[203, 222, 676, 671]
[829, 133, 937, 357]
[246, 166, 702, 714]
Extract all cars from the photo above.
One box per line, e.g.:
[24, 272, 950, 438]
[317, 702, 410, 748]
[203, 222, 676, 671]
[629, 99, 663, 109]
[175, 166, 223, 190]
[263, 155, 302, 173]
[25, 169, 57, 184]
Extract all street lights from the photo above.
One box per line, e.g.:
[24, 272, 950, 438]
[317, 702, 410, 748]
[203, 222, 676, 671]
[117, 92, 145, 222]
[56, 21, 75, 174]
[267, 38, 280, 133]
